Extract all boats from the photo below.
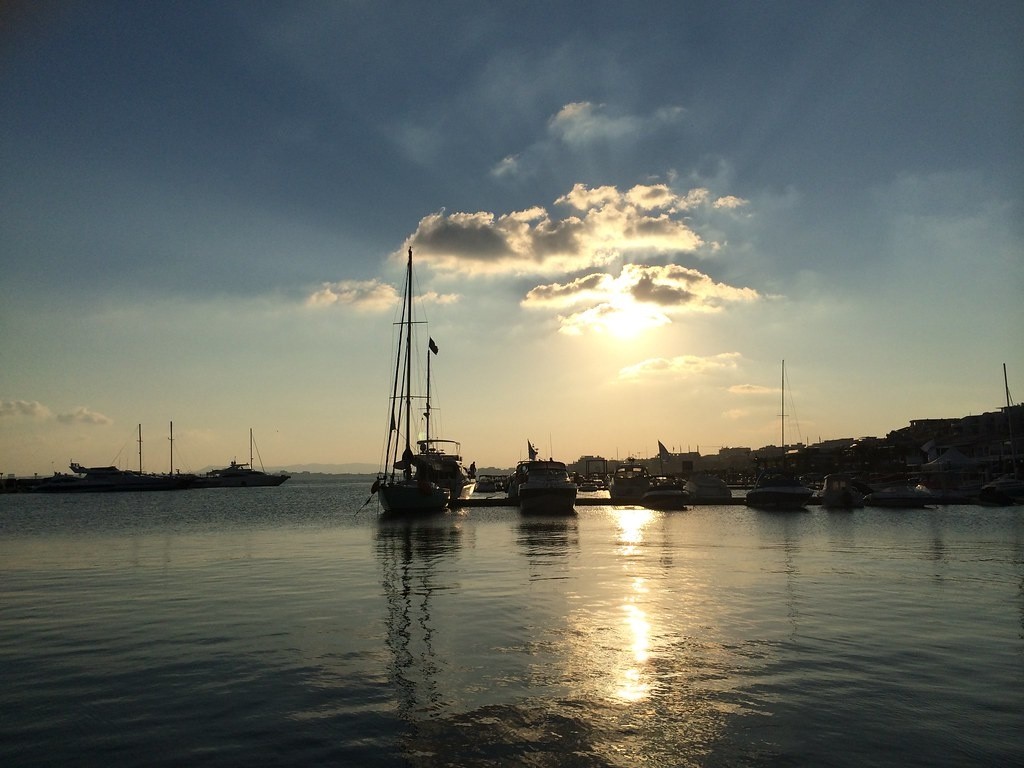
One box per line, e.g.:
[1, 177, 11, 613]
[877, 482, 933, 510]
[608, 463, 652, 500]
[747, 464, 816, 515]
[507, 458, 579, 513]
[813, 472, 878, 511]
[639, 473, 692, 509]
[473, 465, 609, 494]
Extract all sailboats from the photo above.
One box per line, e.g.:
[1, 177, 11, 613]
[34, 419, 200, 495]
[192, 428, 292, 487]
[370, 246, 477, 516]
